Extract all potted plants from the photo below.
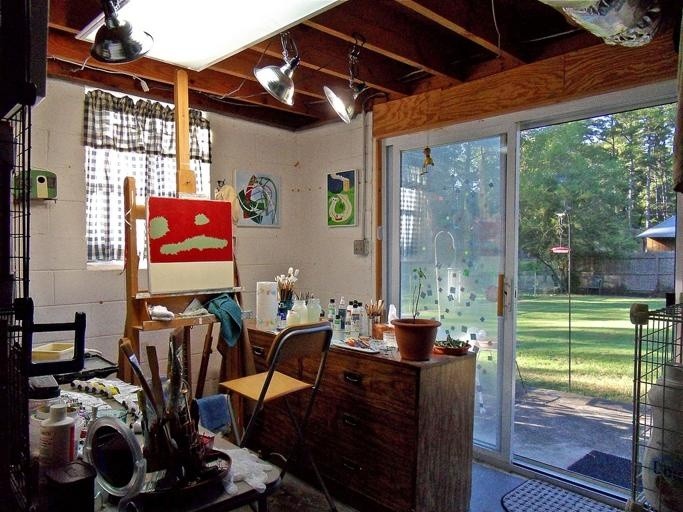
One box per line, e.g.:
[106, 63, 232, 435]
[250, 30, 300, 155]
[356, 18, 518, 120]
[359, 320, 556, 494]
[390, 268, 442, 361]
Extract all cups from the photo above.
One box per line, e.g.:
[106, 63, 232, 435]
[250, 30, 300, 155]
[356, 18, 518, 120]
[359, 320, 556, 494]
[383, 330, 398, 357]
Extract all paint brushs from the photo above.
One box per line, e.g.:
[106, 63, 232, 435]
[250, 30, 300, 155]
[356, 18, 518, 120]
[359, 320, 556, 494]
[275, 267, 300, 301]
[120, 326, 185, 429]
[366, 297, 385, 317]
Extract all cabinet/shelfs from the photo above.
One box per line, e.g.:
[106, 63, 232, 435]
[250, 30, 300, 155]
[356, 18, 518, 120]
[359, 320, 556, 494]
[225, 320, 477, 512]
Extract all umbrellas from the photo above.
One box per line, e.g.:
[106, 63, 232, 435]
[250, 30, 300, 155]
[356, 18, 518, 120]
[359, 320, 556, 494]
[636, 212, 676, 242]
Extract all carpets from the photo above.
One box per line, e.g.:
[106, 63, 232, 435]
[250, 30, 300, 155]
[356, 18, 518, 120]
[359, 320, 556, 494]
[566, 449, 646, 493]
[500, 478, 626, 512]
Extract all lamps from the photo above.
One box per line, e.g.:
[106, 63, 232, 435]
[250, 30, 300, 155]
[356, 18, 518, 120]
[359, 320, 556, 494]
[254, 32, 300, 107]
[90, 1, 154, 65]
[323, 32, 366, 124]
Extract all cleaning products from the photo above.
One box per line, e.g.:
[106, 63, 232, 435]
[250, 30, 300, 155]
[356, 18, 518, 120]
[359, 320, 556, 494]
[329, 296, 368, 336]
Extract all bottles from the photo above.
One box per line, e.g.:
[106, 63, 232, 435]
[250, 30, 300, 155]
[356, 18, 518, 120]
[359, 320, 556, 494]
[326, 296, 370, 337]
[37, 404, 75, 466]
[286, 299, 321, 327]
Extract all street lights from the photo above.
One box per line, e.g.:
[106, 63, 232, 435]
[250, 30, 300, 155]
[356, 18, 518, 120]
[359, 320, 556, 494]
[550, 206, 571, 395]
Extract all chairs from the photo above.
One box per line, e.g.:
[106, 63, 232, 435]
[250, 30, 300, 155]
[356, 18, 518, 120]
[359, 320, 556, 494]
[218, 323, 335, 512]
